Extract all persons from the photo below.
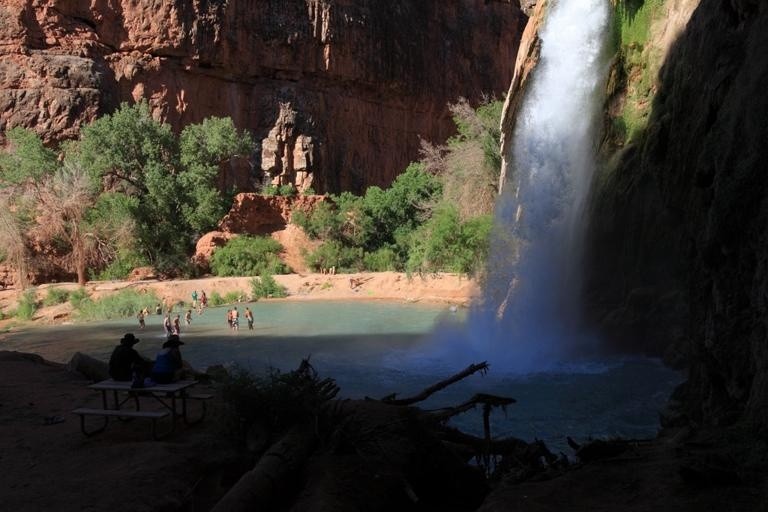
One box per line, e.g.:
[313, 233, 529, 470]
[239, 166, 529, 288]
[109, 329, 153, 381]
[125, 284, 255, 337]
[150, 333, 206, 384]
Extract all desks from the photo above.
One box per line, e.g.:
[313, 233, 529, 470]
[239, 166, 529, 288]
[83, 376, 199, 433]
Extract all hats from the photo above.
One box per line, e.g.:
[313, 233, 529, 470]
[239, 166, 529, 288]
[121, 333, 140, 344]
[162, 334, 184, 347]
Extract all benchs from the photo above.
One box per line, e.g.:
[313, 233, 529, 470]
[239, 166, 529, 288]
[64, 390, 214, 439]
